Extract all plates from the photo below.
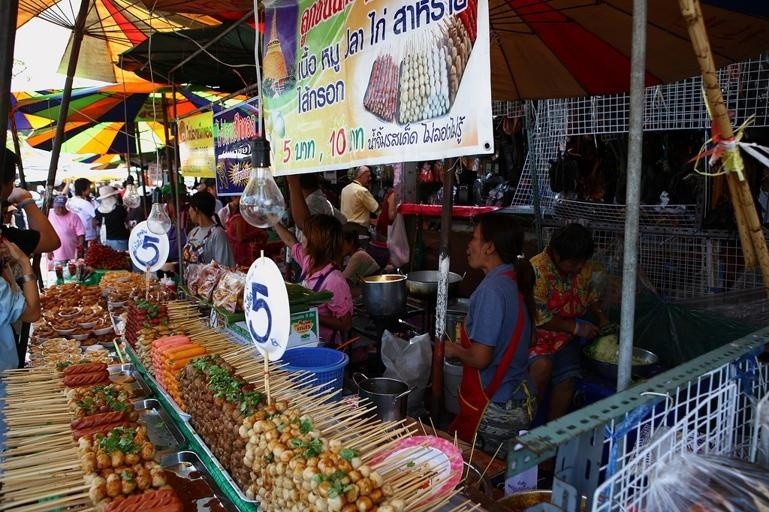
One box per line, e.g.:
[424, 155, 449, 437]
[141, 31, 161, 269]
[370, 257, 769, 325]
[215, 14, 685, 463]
[359, 436, 465, 510]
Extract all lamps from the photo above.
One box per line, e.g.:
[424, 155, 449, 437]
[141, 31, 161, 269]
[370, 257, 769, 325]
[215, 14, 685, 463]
[111, 0, 144, 212]
[143, 2, 175, 238]
[233, 0, 292, 230]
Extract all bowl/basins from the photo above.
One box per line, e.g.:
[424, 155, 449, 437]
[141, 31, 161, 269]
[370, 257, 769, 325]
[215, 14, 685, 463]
[35, 303, 114, 341]
[461, 456, 490, 510]
[582, 341, 660, 380]
[492, 489, 588, 511]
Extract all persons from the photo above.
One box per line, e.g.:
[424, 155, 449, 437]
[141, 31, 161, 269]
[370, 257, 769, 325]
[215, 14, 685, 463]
[0, 165, 611, 460]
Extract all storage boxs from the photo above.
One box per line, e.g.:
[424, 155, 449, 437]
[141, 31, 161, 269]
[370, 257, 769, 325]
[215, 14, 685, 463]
[209, 305, 320, 353]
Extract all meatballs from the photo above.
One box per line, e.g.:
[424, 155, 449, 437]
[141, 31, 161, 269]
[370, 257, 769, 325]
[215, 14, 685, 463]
[64, 383, 140, 422]
[177, 351, 276, 494]
[362, 1, 478, 125]
[239, 399, 404, 512]
[78, 423, 173, 510]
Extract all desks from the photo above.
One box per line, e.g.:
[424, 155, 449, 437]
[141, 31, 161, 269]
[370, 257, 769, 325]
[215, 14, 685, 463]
[570, 368, 688, 489]
[391, 407, 509, 490]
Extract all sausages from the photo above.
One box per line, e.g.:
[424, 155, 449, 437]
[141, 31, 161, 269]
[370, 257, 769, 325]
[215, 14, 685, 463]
[150, 335, 208, 410]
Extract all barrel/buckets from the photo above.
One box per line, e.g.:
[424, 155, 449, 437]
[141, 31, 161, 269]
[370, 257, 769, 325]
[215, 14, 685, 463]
[443, 312, 465, 335]
[363, 273, 408, 328]
[443, 358, 465, 415]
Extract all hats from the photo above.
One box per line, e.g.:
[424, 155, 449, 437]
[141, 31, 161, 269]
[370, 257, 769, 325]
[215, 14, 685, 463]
[54, 195, 67, 207]
[96, 186, 119, 201]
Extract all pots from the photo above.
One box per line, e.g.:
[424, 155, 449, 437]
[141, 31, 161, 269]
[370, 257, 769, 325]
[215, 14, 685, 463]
[355, 269, 410, 318]
[397, 268, 467, 301]
